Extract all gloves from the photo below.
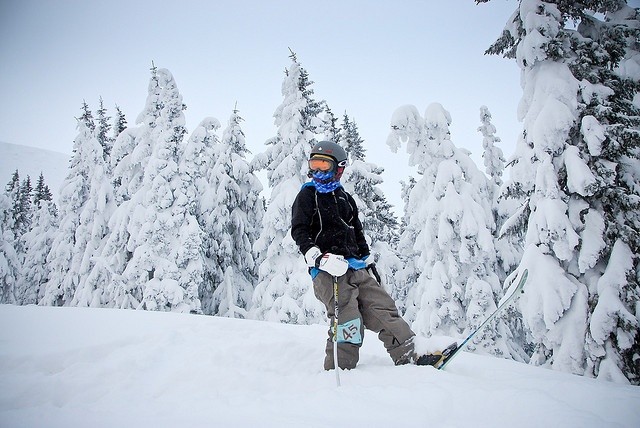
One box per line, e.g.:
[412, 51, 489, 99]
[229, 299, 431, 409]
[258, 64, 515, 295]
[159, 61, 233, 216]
[305, 246, 349, 277]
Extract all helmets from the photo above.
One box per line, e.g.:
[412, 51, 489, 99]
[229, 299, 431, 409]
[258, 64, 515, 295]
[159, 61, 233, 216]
[307, 141, 347, 183]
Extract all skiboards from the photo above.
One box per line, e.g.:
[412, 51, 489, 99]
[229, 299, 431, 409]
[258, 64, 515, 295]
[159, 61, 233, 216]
[437, 269, 528, 372]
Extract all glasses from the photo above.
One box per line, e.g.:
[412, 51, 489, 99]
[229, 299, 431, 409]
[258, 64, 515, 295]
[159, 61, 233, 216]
[308, 155, 337, 172]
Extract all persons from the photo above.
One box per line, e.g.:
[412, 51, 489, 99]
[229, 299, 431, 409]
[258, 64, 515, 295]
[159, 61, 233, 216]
[291, 141, 446, 373]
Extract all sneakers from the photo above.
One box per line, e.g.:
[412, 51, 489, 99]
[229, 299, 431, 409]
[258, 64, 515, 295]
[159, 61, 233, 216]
[413, 355, 441, 369]
